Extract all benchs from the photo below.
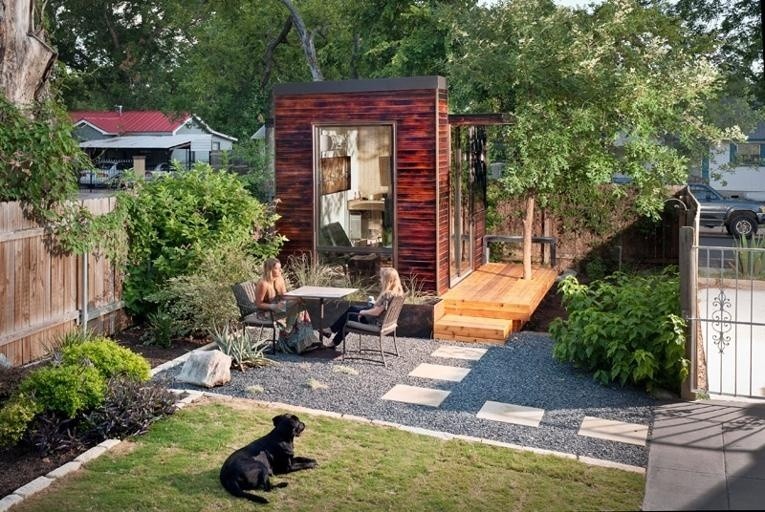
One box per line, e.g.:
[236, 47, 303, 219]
[483, 235, 558, 270]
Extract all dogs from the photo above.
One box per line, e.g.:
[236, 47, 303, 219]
[219, 413, 317, 503]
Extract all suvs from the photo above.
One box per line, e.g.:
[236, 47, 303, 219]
[686, 181, 764, 241]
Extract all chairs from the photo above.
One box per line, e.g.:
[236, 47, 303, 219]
[322, 222, 356, 268]
[343, 290, 411, 368]
[231, 277, 287, 356]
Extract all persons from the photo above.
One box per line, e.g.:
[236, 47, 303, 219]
[254, 257, 326, 354]
[318, 267, 404, 352]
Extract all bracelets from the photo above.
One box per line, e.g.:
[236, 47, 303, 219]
[270, 304, 276, 310]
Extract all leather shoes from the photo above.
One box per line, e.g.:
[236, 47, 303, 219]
[327, 346, 335, 350]
[318, 329, 331, 338]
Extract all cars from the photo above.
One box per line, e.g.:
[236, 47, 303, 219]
[73, 158, 133, 188]
[120, 161, 177, 186]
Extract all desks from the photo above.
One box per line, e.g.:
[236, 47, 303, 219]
[350, 254, 379, 280]
[284, 285, 359, 359]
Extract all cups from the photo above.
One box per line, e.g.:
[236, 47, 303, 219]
[367, 194, 374, 201]
[367, 296, 374, 308]
